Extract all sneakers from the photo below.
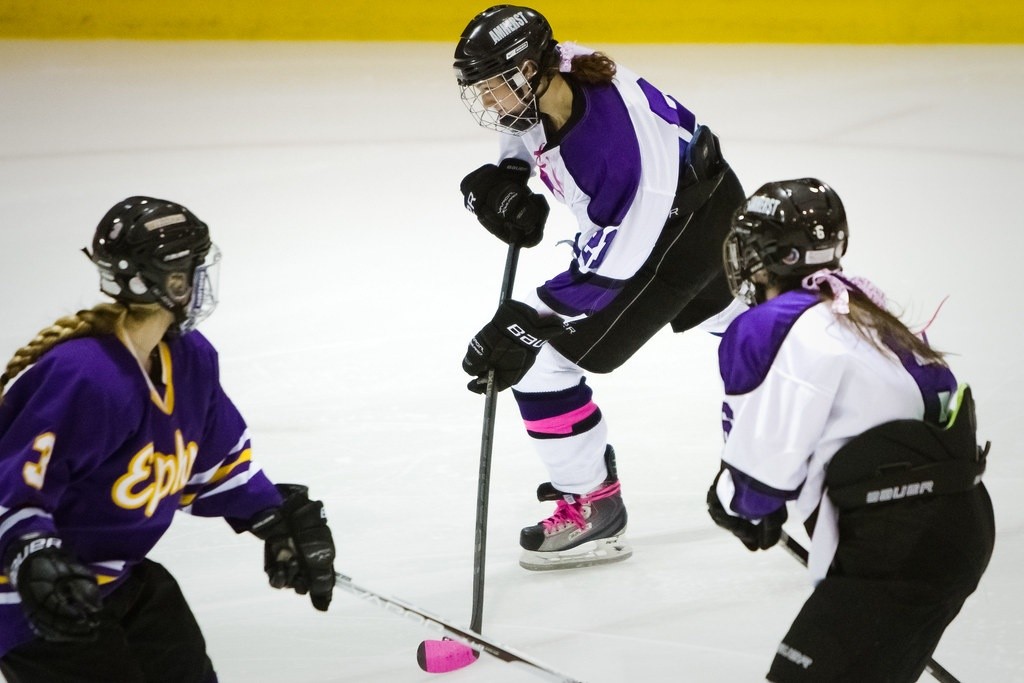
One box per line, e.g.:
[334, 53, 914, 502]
[519, 444, 633, 571]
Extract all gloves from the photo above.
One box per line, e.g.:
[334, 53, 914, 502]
[705, 486, 787, 552]
[247, 484, 336, 612]
[9, 536, 104, 643]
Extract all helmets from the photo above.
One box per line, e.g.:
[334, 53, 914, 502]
[453, 4, 558, 97]
[731, 177, 848, 281]
[460, 158, 550, 248]
[90, 196, 211, 304]
[462, 299, 565, 394]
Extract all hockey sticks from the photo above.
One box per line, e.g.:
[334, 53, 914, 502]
[275, 541, 582, 682]
[751, 515, 963, 683]
[416, 240, 521, 674]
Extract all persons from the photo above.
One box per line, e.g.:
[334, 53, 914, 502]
[452, 4, 754, 571]
[0, 196, 336, 683]
[706, 178, 996, 683]
